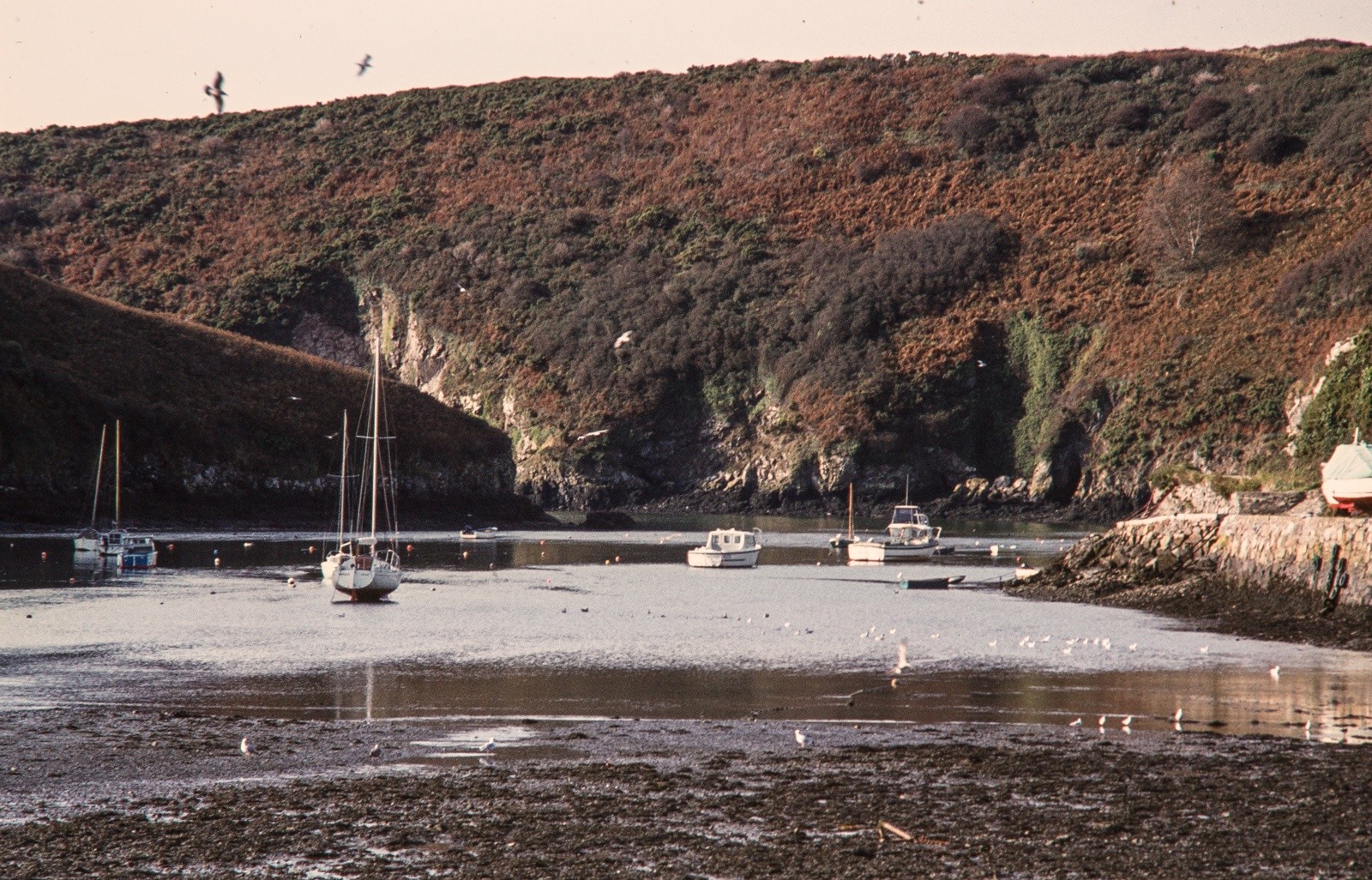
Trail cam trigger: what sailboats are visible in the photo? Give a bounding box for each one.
[73,424,107,551]
[321,326,403,601]
[74,550,100,565]
[99,420,127,554]
[98,554,123,569]
[828,482,862,551]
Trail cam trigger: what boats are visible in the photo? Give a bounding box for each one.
[1319,426,1372,514]
[688,526,764,569]
[847,535,940,562]
[885,473,942,542]
[898,577,949,591]
[122,533,158,554]
[122,552,157,571]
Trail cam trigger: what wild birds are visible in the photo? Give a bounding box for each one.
[25,528,1314,757]
[613,331,632,347]
[356,54,372,74]
[205,70,228,114]
